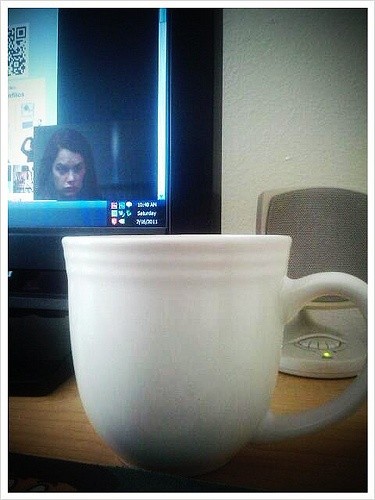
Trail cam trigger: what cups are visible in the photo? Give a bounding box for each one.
[59,233,367,480]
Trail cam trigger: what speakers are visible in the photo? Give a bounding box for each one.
[254,186,368,378]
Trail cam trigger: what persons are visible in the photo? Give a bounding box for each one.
[35,127,102,202]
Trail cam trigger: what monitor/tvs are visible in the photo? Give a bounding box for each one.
[8,8,224,313]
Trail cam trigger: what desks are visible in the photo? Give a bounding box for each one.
[8,369,368,493]
